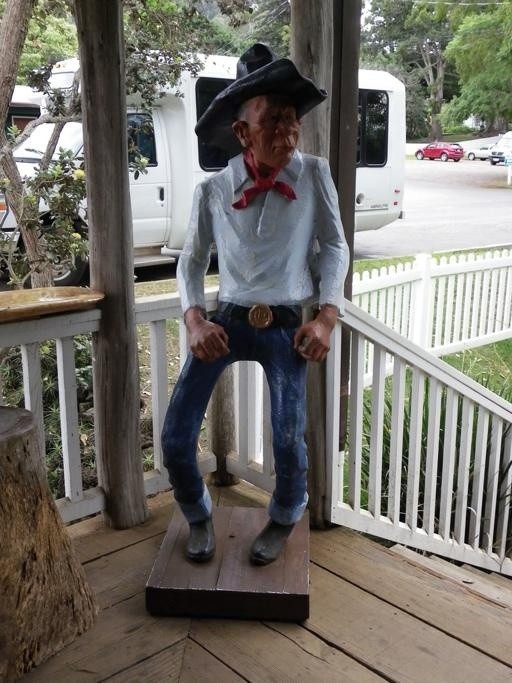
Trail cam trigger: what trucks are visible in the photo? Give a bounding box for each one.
[0,50,409,289]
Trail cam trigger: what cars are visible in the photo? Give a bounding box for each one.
[463,144,494,161]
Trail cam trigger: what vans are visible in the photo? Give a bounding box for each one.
[488,131,512,166]
[414,142,464,162]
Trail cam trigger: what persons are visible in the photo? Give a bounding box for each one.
[160,40,350,564]
